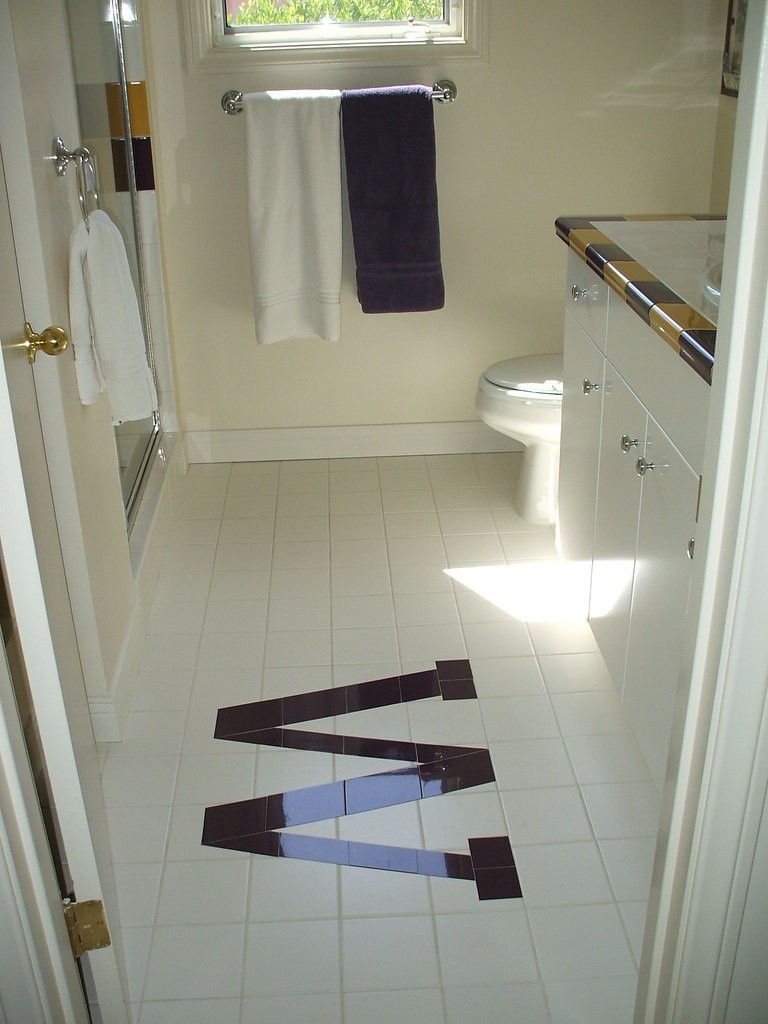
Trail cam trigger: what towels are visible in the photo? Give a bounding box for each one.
[241,88,342,344]
[69,208,160,428]
[340,84,446,313]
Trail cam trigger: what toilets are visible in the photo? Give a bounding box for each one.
[477,352,564,526]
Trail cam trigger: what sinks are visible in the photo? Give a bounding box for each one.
[699,259,722,307]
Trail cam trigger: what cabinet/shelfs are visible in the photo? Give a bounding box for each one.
[554,243,712,794]
